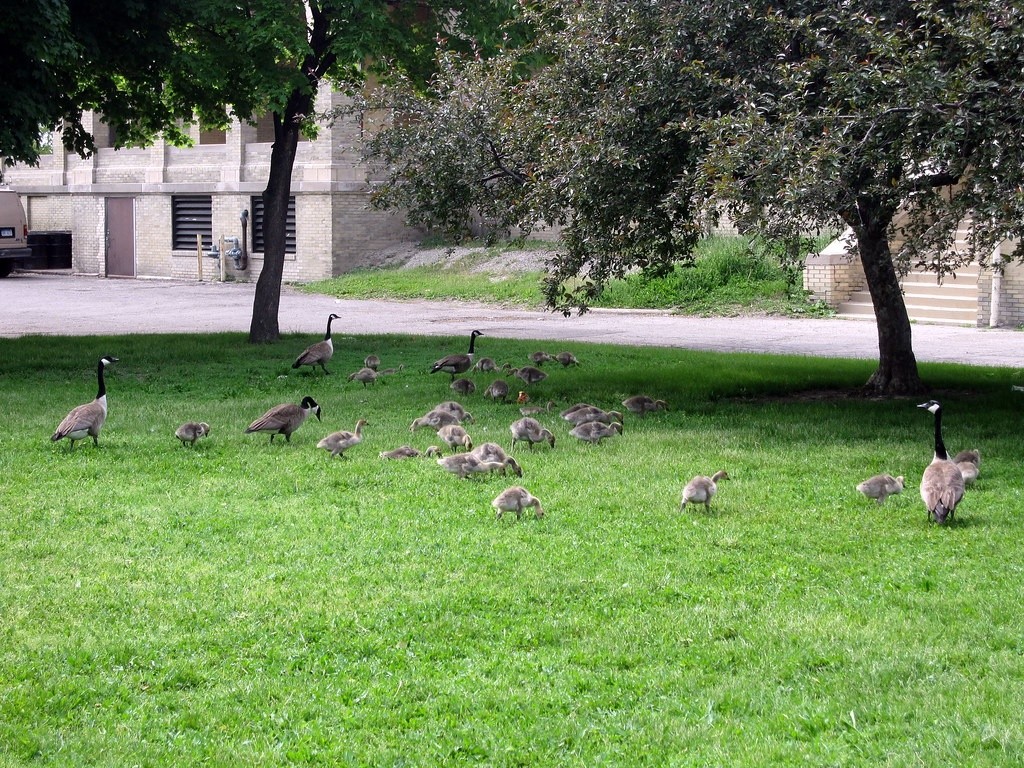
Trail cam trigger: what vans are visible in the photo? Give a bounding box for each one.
[0,189,32,277]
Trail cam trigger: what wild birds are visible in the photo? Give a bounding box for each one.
[917,400,965,525]
[856,474,905,503]
[679,470,731,512]
[317,419,369,460]
[492,486,544,520]
[380,329,671,478]
[175,422,210,447]
[953,449,980,465]
[347,369,378,388]
[51,355,120,450]
[244,396,321,442]
[292,314,341,375]
[377,364,404,375]
[956,461,979,488]
[364,355,380,370]
